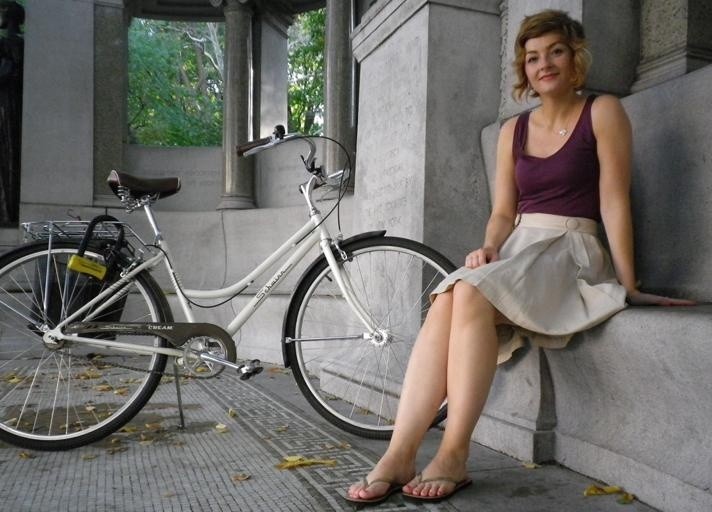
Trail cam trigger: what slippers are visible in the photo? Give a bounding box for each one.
[343,474,407,507]
[402,471,473,504]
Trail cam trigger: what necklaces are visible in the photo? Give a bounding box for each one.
[541,100,571,136]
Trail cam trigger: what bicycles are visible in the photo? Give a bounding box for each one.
[0,125,459,453]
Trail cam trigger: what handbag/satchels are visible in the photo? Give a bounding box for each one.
[28,253,128,342]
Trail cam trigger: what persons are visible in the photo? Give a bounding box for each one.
[345,10,698,502]
